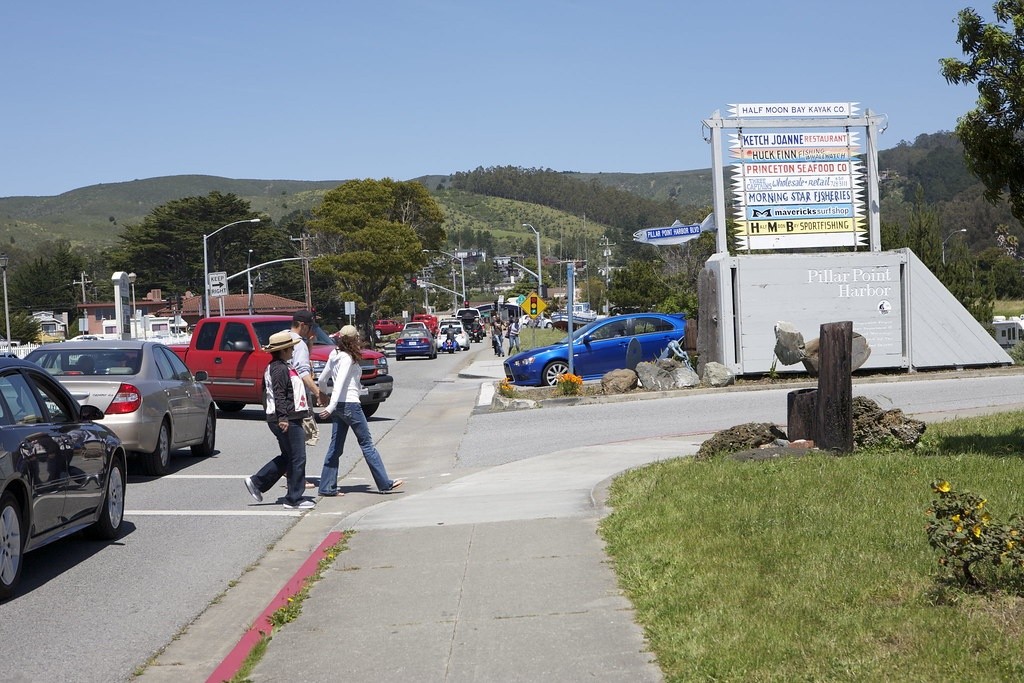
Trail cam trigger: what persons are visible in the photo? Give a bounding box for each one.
[471,318,482,343]
[444,324,461,351]
[317,325,403,496]
[490,315,506,357]
[284,310,327,490]
[611,323,625,338]
[509,316,513,324]
[506,318,521,356]
[245,332,316,509]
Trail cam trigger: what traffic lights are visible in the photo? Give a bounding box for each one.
[411,277,417,289]
[522,291,548,319]
[464,300,470,308]
[493,260,501,275]
[506,260,513,272]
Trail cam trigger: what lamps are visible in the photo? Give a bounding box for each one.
[701,124,711,145]
[868,112,889,134]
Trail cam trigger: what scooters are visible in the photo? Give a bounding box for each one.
[473,329,482,343]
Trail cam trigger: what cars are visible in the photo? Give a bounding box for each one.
[66,334,111,343]
[503,312,689,387]
[404,322,428,330]
[413,302,488,349]
[375,320,406,337]
[396,329,439,361]
[20,342,221,477]
[0,354,126,596]
[517,314,554,330]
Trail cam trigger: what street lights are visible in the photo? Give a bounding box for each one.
[201,218,266,320]
[942,228,967,266]
[246,249,258,316]
[128,272,140,340]
[0,252,14,353]
[523,223,544,298]
[423,248,467,309]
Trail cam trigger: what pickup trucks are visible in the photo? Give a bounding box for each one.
[0,336,21,349]
[106,316,393,421]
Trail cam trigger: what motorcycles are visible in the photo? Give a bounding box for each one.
[443,338,460,354]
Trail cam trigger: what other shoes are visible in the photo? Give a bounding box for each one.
[305,481,315,488]
[318,490,346,496]
[382,479,401,493]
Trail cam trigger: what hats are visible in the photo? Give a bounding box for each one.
[261,331,301,352]
[293,310,318,324]
[339,325,358,339]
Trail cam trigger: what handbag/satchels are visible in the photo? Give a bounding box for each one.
[301,416,319,446]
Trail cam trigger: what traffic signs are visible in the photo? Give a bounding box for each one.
[208,271,228,298]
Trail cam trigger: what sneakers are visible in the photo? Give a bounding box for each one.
[283,500,315,509]
[244,477,263,502]
